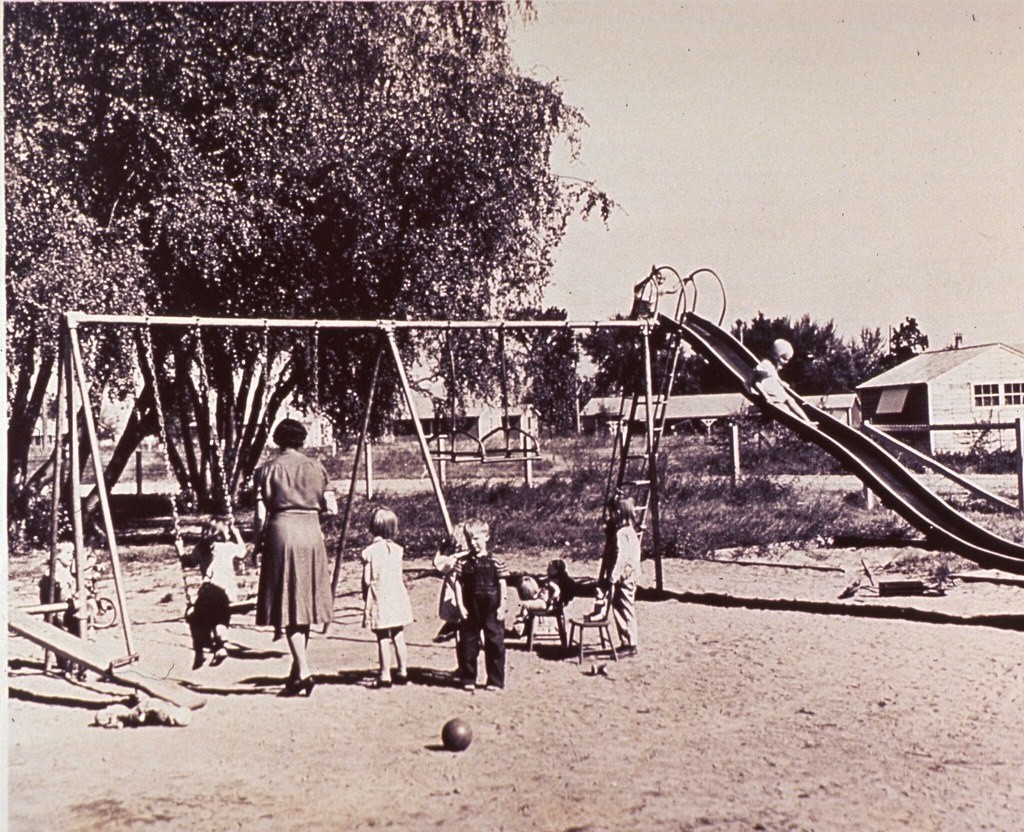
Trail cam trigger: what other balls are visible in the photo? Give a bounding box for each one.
[441,718,473,751]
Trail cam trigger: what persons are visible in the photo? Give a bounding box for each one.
[173,515,247,671]
[436,492,643,692]
[38,540,97,646]
[250,419,339,694]
[359,508,413,688]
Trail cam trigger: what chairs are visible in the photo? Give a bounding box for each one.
[527,572,568,652]
[567,584,619,665]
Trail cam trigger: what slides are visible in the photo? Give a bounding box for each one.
[657,309,1024,573]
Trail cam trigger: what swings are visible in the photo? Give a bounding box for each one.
[141,325,257,624]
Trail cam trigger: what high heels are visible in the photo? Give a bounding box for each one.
[278,675,316,697]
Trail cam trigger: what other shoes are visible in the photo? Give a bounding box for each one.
[391,668,409,686]
[208,649,227,666]
[366,672,392,687]
[610,645,637,660]
[192,652,206,670]
